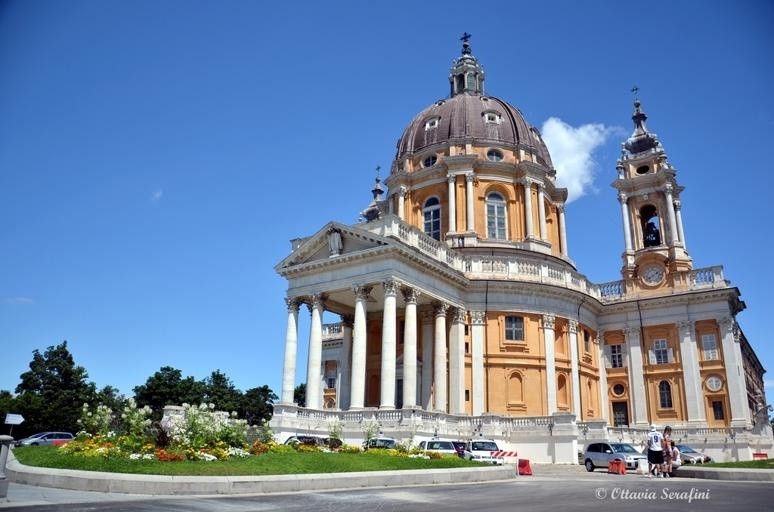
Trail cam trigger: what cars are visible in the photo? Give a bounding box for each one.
[417,440,504,466]
[283,435,396,449]
[584,443,711,472]
[16,432,74,447]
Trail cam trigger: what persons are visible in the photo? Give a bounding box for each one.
[647,424,681,478]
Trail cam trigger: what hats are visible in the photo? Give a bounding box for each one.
[651,424,657,432]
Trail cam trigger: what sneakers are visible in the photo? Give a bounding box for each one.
[647,470,675,478]
